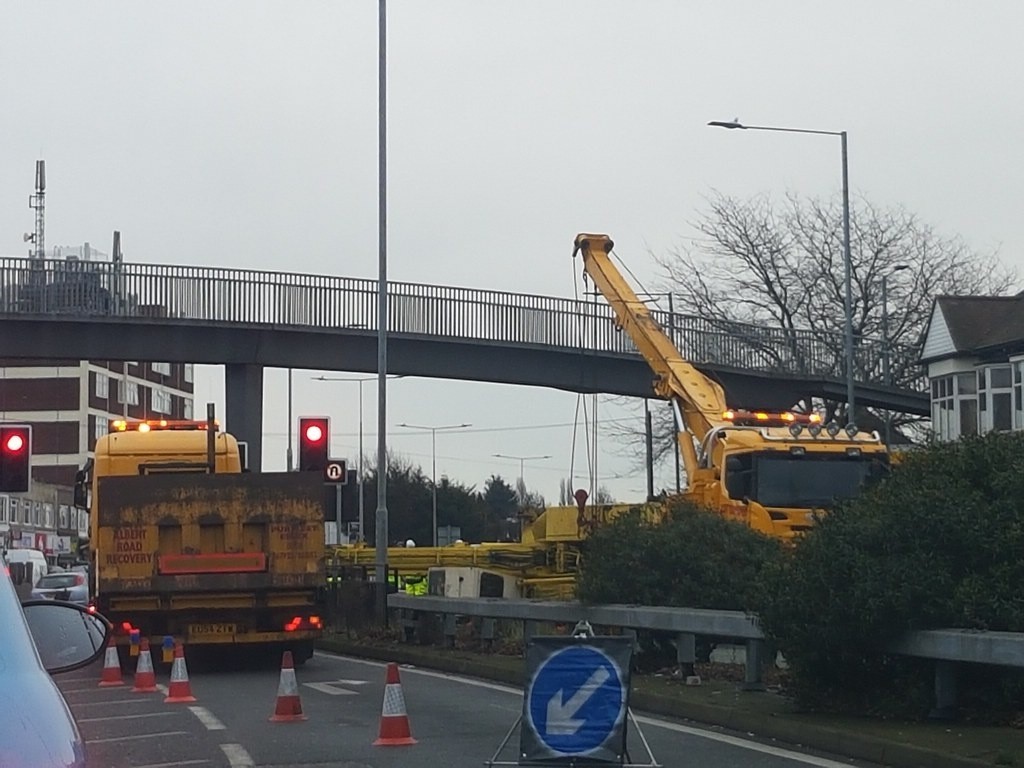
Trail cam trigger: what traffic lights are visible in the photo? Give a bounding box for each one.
[1,425,31,494]
[298,417,329,485]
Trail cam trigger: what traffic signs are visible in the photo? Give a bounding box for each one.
[525,635,630,767]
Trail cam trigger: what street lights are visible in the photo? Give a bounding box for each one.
[711,116,860,433]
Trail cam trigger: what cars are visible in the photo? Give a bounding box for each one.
[0,526,112,768]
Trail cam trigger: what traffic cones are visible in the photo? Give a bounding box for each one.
[371,664,419,745]
[268,650,308,722]
[162,639,198,702]
[99,634,123,688]
[130,639,158,694]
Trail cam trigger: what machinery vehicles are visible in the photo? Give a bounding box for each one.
[334,232,903,621]
[77,411,327,670]
